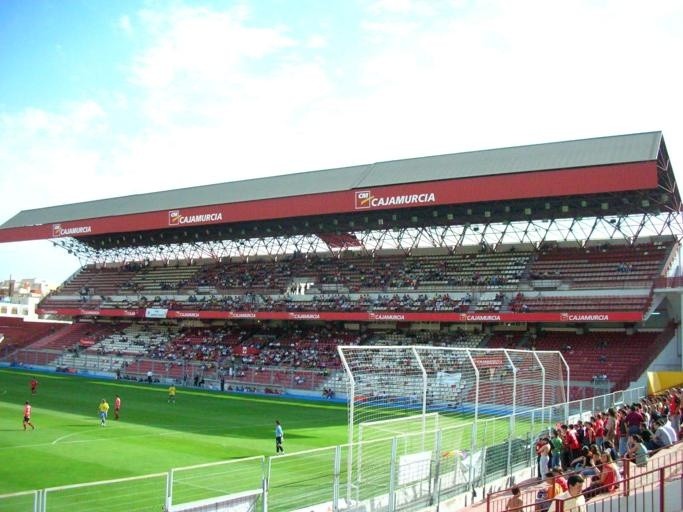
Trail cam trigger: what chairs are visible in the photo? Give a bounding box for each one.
[28,244,669,404]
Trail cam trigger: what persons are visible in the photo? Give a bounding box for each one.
[97,398,110,426]
[48,242,642,410]
[29,376,39,400]
[21,402,36,431]
[113,393,122,419]
[274,419,285,456]
[505,385,683,512]
